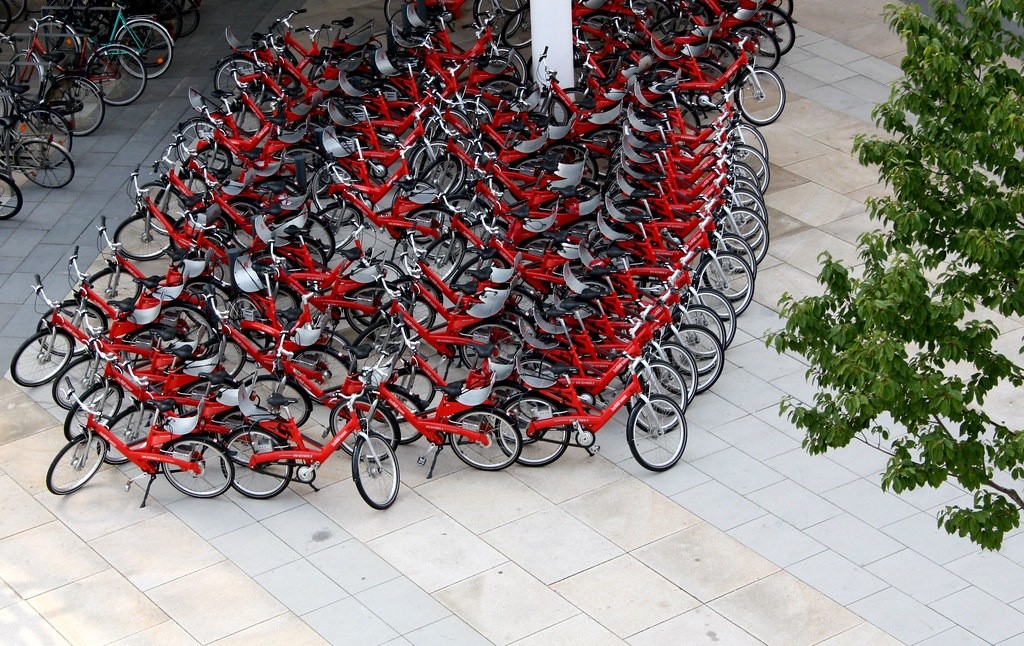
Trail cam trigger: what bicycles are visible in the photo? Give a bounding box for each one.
[0,1,803,511]
